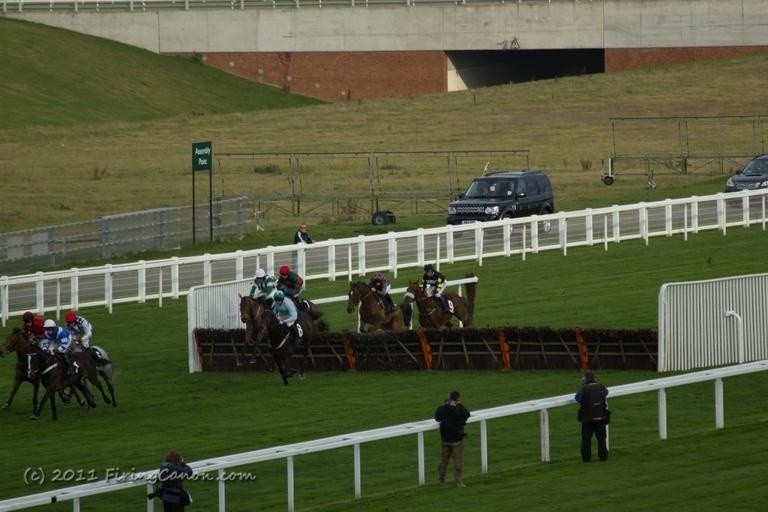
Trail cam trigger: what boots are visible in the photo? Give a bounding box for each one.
[85,348,101,362]
[439,294,450,313]
[61,353,75,375]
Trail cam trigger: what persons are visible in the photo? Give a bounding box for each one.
[23,310,93,374]
[575,371,611,463]
[251,264,447,333]
[158,450,193,512]
[434,390,471,487]
[292,224,313,273]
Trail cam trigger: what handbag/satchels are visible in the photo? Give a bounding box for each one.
[577,406,611,425]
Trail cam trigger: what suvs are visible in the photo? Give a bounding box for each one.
[441,167,555,237]
[722,152,767,208]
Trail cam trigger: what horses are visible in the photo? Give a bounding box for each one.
[259,304,312,388]
[244,308,323,345]
[81,341,114,411]
[404,278,470,329]
[1,326,44,419]
[346,280,406,333]
[236,292,317,324]
[22,339,112,420]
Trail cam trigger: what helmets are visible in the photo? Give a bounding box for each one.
[424,264,434,271]
[65,312,77,324]
[256,268,266,278]
[372,272,384,283]
[43,319,57,328]
[279,266,290,275]
[274,290,284,301]
[23,312,33,323]
